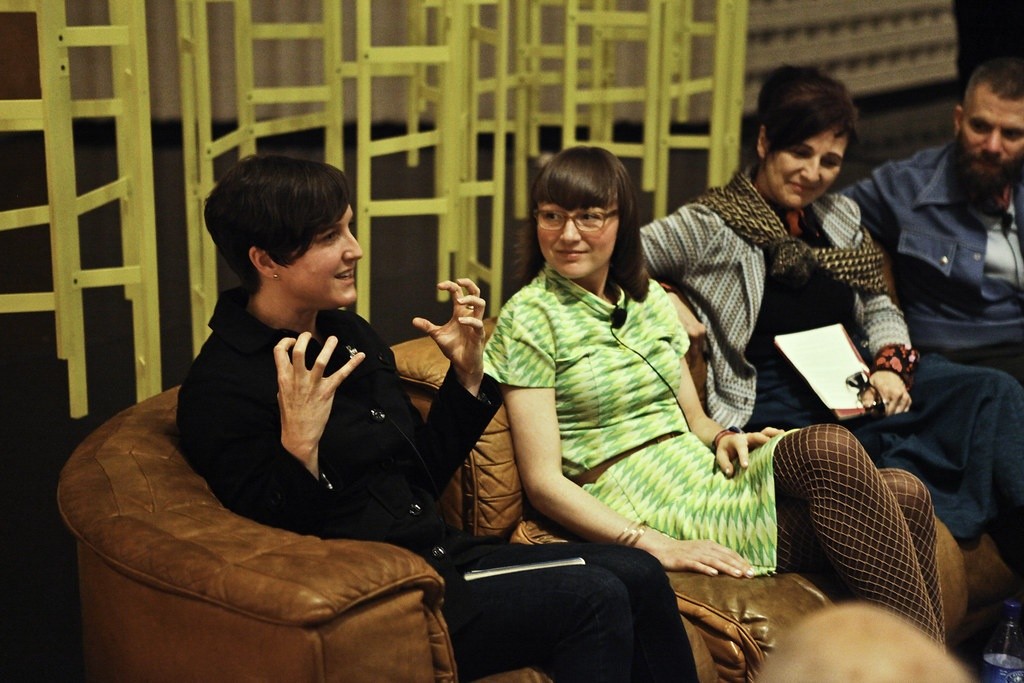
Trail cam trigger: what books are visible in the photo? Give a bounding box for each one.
[774,323,886,421]
[464,556,586,581]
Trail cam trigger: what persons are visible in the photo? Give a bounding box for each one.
[177,156,699,683]
[483,146,947,653]
[641,66,1024,575]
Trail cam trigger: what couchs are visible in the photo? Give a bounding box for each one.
[56,245,1019,683]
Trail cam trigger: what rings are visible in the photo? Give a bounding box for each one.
[466,305,474,309]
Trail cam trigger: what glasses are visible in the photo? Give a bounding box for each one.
[532,209,620,231]
[845,372,885,420]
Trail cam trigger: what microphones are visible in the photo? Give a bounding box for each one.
[609,308,628,329]
[1000,212,1013,237]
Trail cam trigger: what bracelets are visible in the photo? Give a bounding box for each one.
[712,425,741,449]
[616,520,647,547]
